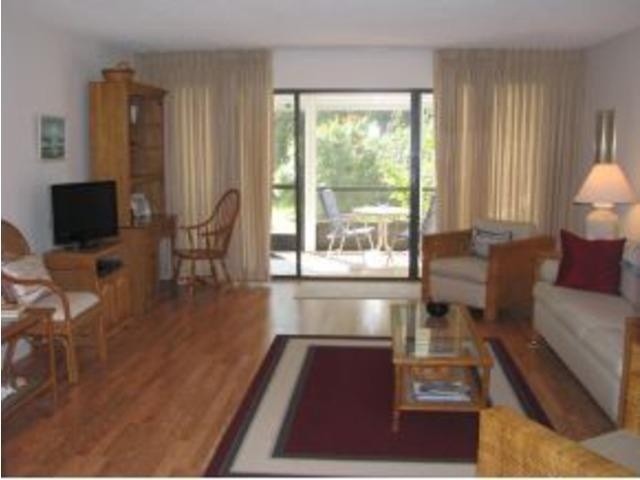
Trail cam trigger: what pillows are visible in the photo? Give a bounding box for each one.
[4,252,55,309]
[554,226,626,297]
[466,227,513,262]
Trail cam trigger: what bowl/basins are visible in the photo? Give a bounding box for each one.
[426,303,449,316]
[103,68,134,81]
[426,317,448,329]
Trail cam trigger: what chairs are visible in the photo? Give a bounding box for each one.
[385,195,440,267]
[317,187,374,263]
[2,217,107,381]
[170,189,243,296]
[477,314,640,477]
[419,216,558,321]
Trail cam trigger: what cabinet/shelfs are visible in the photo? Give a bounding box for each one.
[45,237,135,344]
[87,80,169,226]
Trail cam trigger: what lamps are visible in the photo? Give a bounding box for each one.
[571,164,636,237]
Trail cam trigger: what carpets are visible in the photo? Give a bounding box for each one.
[196,333,555,477]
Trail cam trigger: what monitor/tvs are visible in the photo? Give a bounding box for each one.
[51,180,118,249]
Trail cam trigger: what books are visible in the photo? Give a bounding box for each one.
[408,340,472,402]
[2,302,26,321]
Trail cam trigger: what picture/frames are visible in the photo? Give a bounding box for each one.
[36,112,68,164]
[128,192,152,218]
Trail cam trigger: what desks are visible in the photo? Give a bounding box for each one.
[112,213,178,308]
[353,202,413,264]
[0,304,58,427]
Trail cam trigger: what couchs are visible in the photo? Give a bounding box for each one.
[523,232,640,428]
